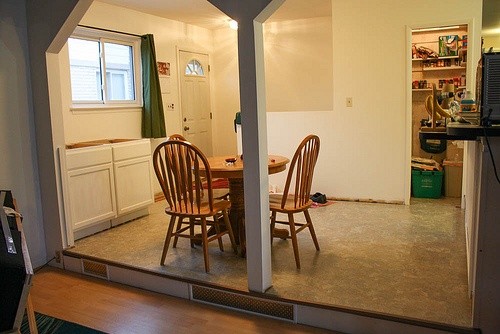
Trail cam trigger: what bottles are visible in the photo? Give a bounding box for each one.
[461,75,466,86]
[412,44,416,59]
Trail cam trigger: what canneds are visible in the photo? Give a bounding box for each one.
[423,60,447,68]
[415,80,427,89]
[439,79,453,88]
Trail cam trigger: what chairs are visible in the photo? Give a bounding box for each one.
[270,135,320,270]
[153,140,237,273]
[164,134,226,252]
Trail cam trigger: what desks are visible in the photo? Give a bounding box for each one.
[190,153,289,245]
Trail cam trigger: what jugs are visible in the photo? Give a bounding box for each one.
[234,112,242,157]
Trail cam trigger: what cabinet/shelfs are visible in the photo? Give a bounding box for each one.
[412,24,469,162]
[66,138,154,241]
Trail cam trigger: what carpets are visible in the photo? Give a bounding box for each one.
[20,307,109,334]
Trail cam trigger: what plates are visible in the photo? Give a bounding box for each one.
[226,158,237,163]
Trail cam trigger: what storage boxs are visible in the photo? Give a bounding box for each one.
[413,168,444,199]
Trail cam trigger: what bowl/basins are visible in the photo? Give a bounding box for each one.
[461,99,474,111]
[426,122,442,127]
